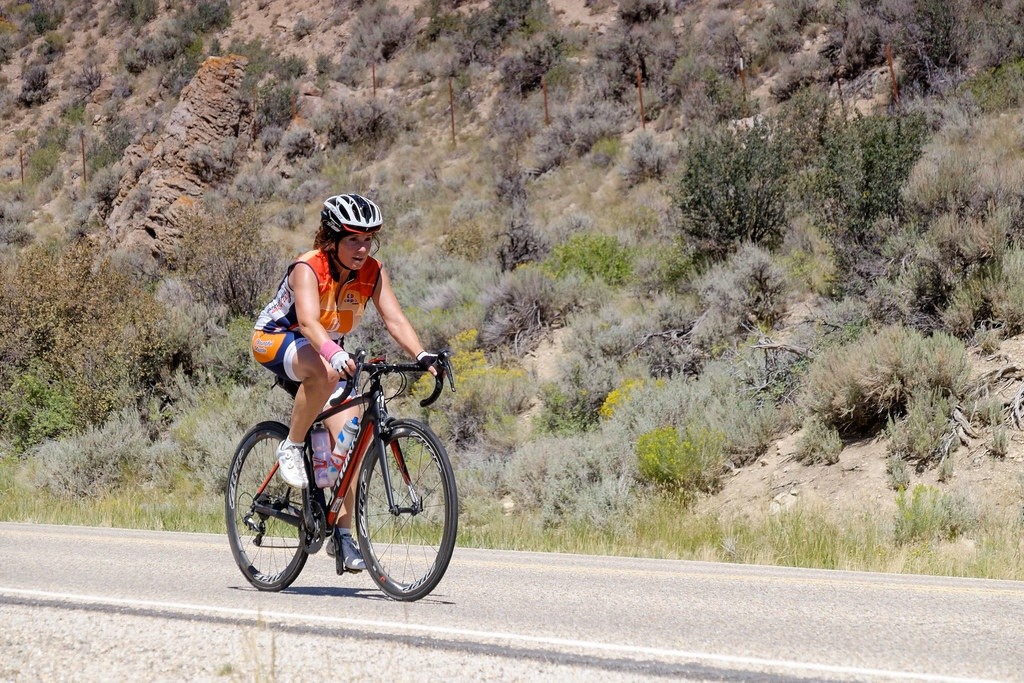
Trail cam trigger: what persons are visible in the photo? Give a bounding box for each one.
[250,193,447,570]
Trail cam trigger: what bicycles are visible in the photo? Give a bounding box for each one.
[225,348,458,602]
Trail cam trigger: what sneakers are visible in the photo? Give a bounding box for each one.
[326,533,373,570]
[275,435,309,489]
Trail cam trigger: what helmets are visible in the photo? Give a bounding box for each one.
[320,193,384,235]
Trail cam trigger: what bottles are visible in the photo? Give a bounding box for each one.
[311,423,335,488]
[326,417,360,479]
